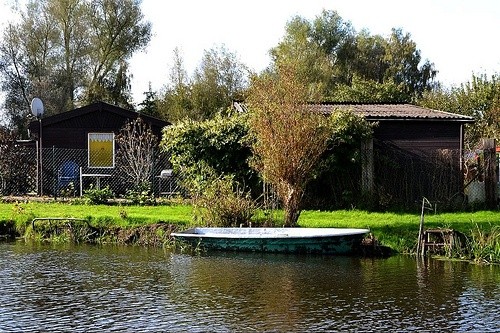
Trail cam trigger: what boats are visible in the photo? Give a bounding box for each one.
[170,225,371,255]
